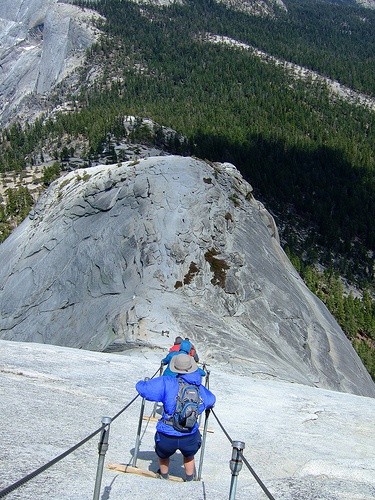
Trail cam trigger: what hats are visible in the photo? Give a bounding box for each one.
[169,353,199,374]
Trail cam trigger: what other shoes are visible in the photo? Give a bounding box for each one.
[181,472,194,482]
[156,468,169,480]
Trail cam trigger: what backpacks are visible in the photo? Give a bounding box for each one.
[159,374,203,433]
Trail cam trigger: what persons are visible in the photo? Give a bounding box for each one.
[136,354,216,482]
[162,341,206,377]
[170,337,195,356]
